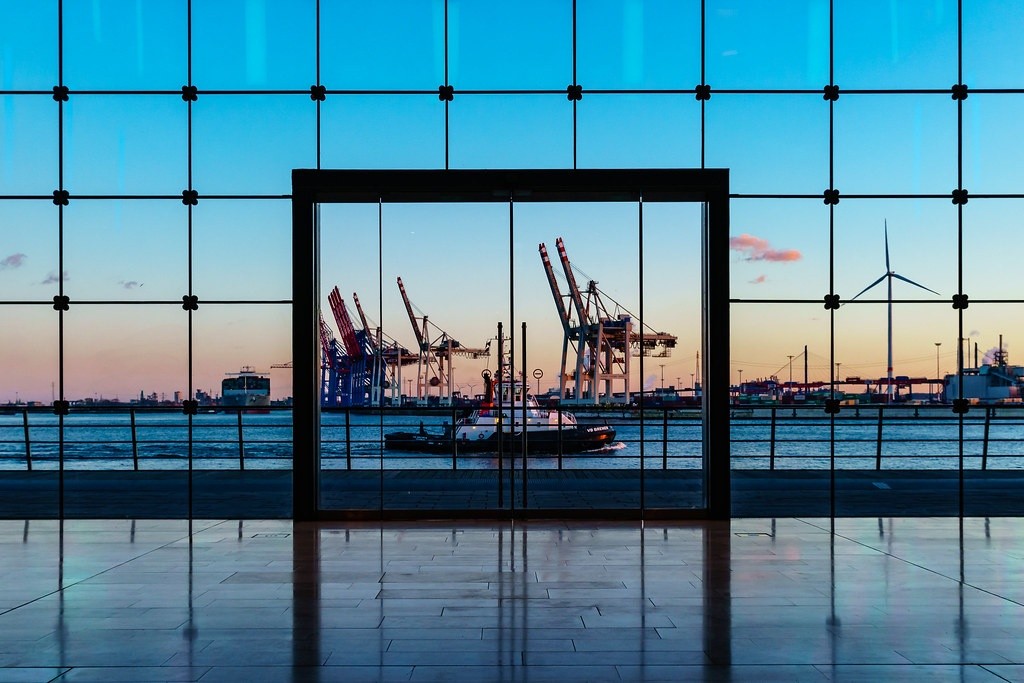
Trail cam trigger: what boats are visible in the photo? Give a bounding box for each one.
[385,335,616,456]
[221,365,270,414]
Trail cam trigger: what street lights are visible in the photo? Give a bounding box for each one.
[659,365,666,396]
[935,343,941,393]
[690,373,695,396]
[676,377,680,389]
[836,363,842,391]
[737,370,743,383]
[787,355,795,395]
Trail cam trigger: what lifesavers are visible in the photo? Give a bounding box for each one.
[478,434,484,439]
